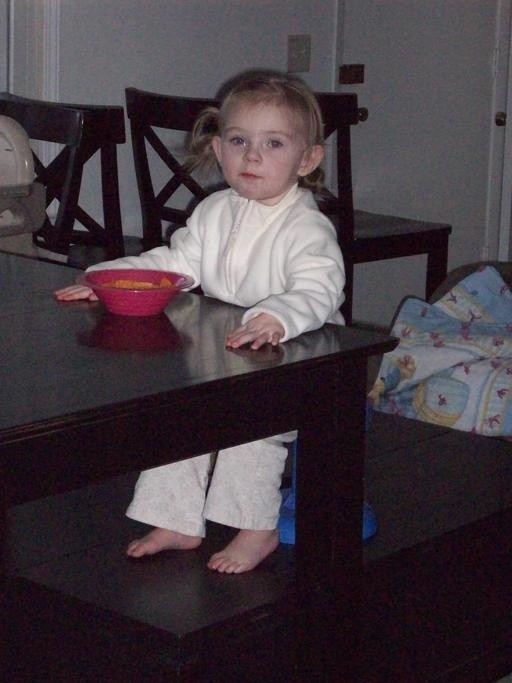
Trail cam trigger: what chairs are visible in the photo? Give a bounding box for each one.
[0,88,452,331]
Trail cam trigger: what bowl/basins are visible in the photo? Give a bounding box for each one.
[74,268,195,318]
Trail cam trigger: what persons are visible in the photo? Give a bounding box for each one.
[51,67,350,577]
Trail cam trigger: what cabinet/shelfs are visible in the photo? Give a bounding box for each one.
[0,401,512,683]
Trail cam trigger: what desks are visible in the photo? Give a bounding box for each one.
[1,254,402,681]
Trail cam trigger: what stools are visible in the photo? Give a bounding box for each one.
[275,405,381,549]
